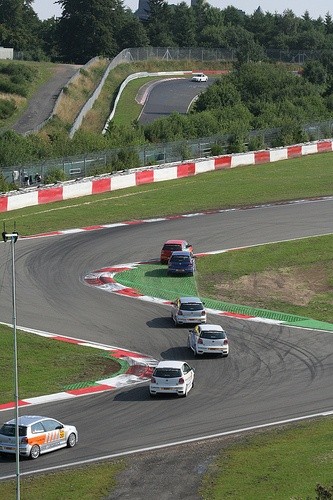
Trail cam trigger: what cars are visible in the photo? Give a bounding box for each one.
[149,360,195,398]
[0,415,78,460]
[170,296,206,327]
[168,251,196,276]
[161,240,192,265]
[187,324,229,358]
[192,73,209,82]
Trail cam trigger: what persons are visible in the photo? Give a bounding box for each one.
[22,168,28,182]
[29,175,32,186]
[34,173,42,182]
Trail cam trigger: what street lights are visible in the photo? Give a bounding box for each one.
[2,231,21,500]
